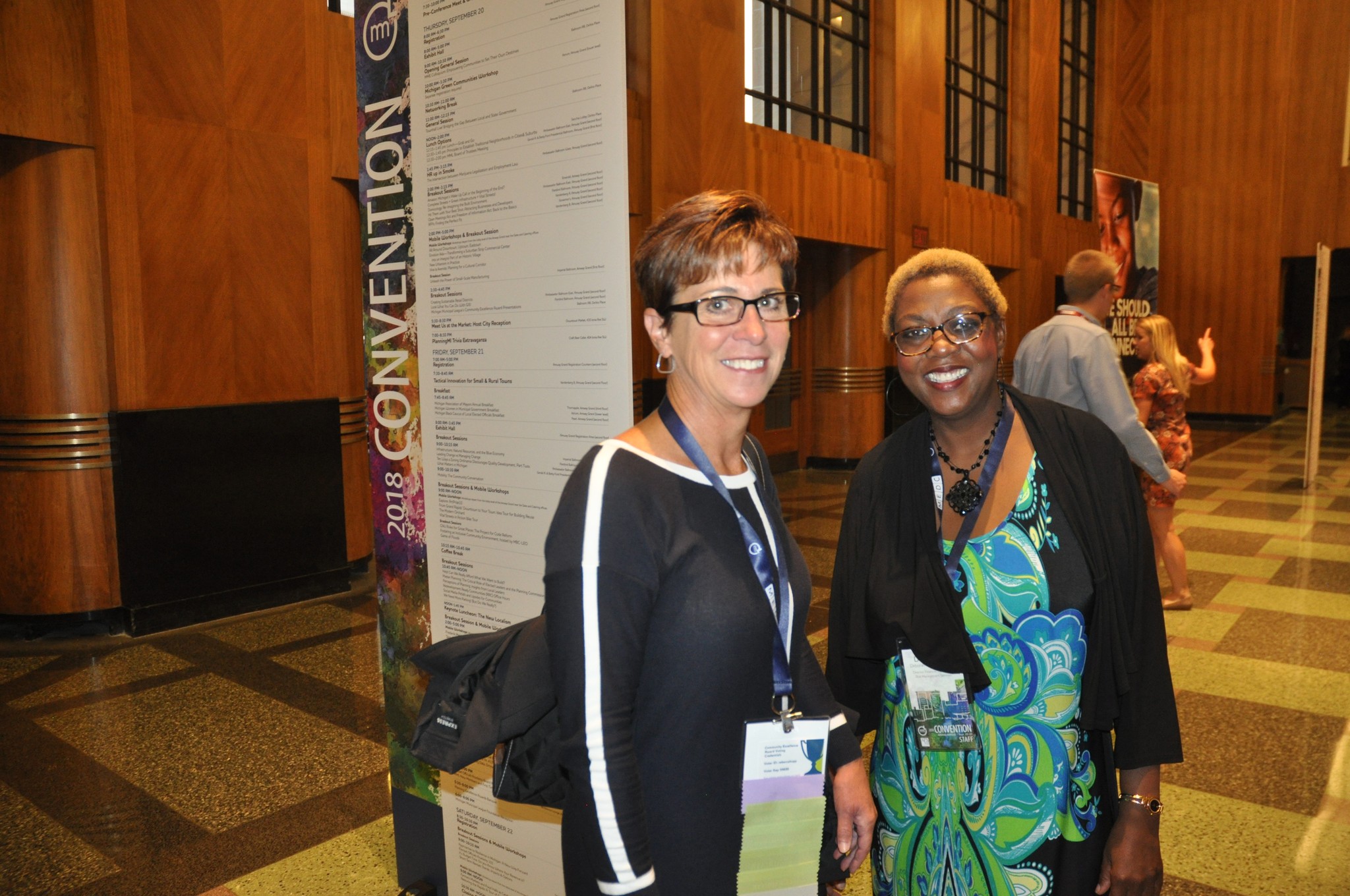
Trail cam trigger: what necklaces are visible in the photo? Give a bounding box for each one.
[928,396,1005,516]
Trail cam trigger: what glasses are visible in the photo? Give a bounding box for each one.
[669,292,800,327]
[1111,283,1121,292]
[890,311,997,356]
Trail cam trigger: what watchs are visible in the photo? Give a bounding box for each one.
[1118,794,1163,815]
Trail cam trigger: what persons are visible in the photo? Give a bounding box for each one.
[1012,249,1188,500]
[826,247,1184,896]
[1093,171,1158,382]
[1130,314,1217,611]
[542,188,878,896]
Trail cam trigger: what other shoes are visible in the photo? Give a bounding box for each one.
[1162,596,1192,611]
